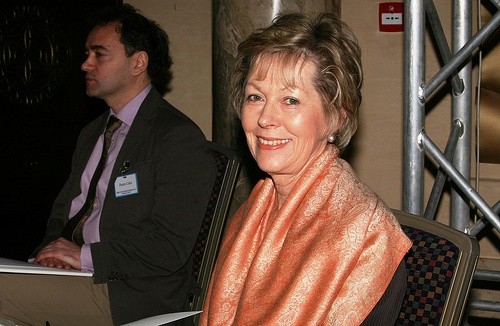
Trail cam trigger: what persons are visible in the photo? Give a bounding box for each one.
[1,3,218,326]
[196,10,414,326]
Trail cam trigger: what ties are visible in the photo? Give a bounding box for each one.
[62,115,123,245]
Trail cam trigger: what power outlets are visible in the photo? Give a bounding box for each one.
[378,1,404,32]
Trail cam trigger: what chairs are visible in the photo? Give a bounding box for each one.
[184,141,244,326]
[387,208,482,326]
